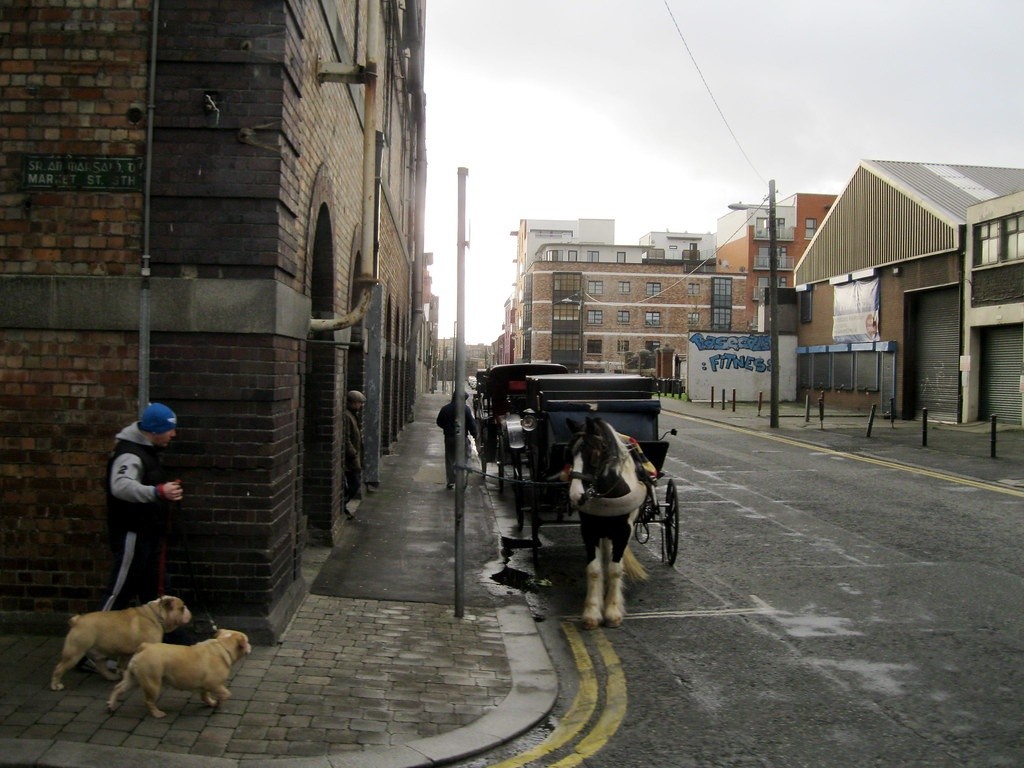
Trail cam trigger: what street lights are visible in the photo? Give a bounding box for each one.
[728,203,780,429]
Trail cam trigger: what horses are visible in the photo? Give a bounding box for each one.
[565,416,650,630]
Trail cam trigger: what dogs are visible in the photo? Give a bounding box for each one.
[106,629,251,718]
[50,596,192,690]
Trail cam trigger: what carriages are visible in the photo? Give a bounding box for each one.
[471,359,679,631]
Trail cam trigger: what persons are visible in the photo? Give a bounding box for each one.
[438,390,481,489]
[81,404,179,681]
[343,391,367,518]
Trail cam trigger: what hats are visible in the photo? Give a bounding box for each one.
[140,402,178,432]
[346,390,368,402]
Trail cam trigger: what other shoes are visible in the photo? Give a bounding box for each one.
[84,657,121,680]
